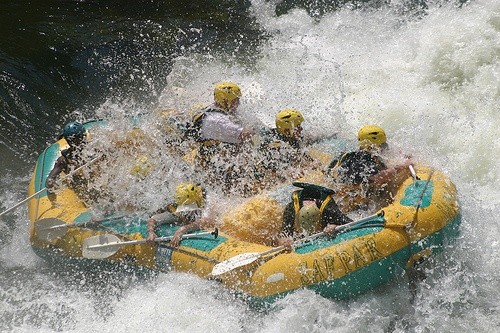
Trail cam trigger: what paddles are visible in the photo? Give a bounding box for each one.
[81,227,218,259]
[33,211,139,242]
[0,153,103,217]
[409,165,420,181]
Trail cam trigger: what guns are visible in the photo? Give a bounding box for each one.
[212,209,385,275]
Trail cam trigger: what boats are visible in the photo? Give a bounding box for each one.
[28,103,461,315]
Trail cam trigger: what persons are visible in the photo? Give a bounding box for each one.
[280,181,353,252]
[251,108,338,183]
[180,82,256,167]
[146,181,212,246]
[333,125,415,214]
[45,121,106,202]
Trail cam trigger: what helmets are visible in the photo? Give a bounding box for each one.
[173,184,203,207]
[298,204,321,233]
[358,125,385,149]
[213,82,241,107]
[63,121,86,141]
[275,109,304,138]
